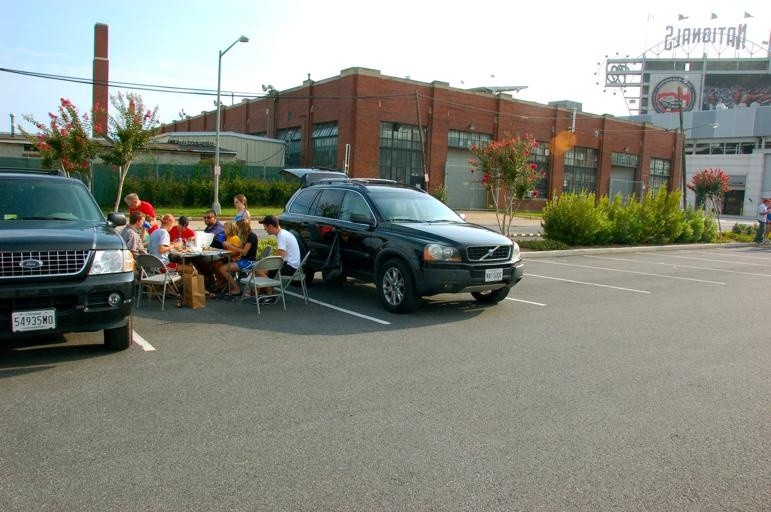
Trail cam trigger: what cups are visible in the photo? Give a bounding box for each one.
[218,234,225,240]
[143,221,150,228]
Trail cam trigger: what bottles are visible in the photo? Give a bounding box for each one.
[176,292,182,307]
[177,237,184,249]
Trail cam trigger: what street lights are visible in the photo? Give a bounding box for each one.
[675,86,720,212]
[209,34,251,214]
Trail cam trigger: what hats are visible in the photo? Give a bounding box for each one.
[259,214,279,225]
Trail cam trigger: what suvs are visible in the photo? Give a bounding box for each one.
[1,165,134,355]
[275,164,526,313]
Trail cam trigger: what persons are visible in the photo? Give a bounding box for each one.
[755,199,771,242]
[120,193,301,305]
[707,86,761,110]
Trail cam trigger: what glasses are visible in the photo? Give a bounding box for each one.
[202,215,216,220]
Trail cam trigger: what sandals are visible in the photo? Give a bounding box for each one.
[224,292,242,297]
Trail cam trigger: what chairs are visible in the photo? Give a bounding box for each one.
[128,235,311,316]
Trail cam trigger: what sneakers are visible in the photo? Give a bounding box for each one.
[254,298,273,305]
